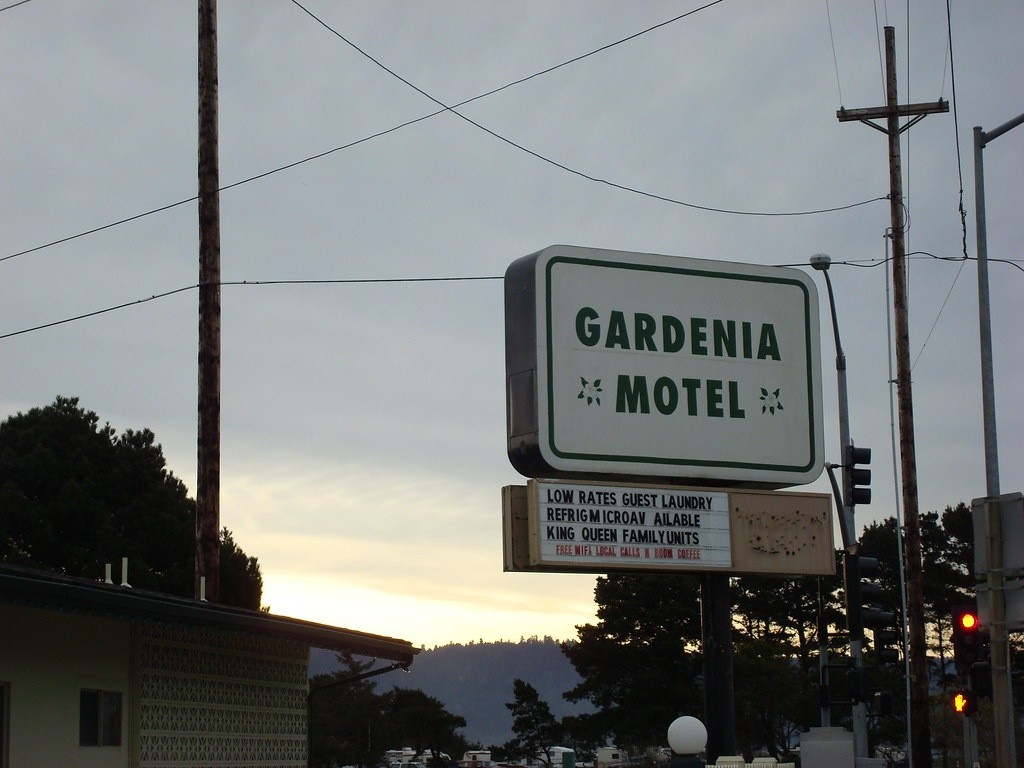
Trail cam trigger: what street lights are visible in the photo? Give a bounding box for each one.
[810,253,877,680]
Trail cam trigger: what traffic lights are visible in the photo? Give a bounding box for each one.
[873,612,900,663]
[952,691,970,714]
[952,606,980,673]
[842,554,884,628]
[842,444,871,505]
